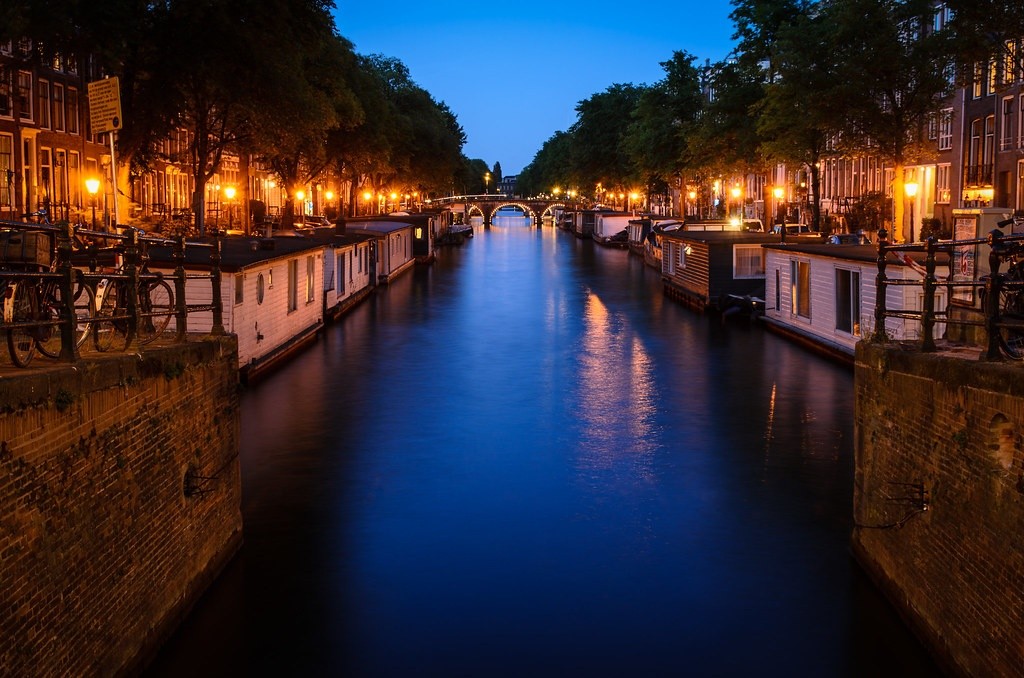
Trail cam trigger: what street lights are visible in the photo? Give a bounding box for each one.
[86,180,100,231]
[297,191,303,223]
[226,187,235,230]
[905,183,919,244]
[774,187,783,224]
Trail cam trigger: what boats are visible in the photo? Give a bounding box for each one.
[624,215,685,270]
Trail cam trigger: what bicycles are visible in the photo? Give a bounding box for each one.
[93,224,174,353]
[980,210,1024,361]
[0,210,96,369]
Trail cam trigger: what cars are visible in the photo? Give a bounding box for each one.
[773,224,809,234]
[831,234,871,245]
